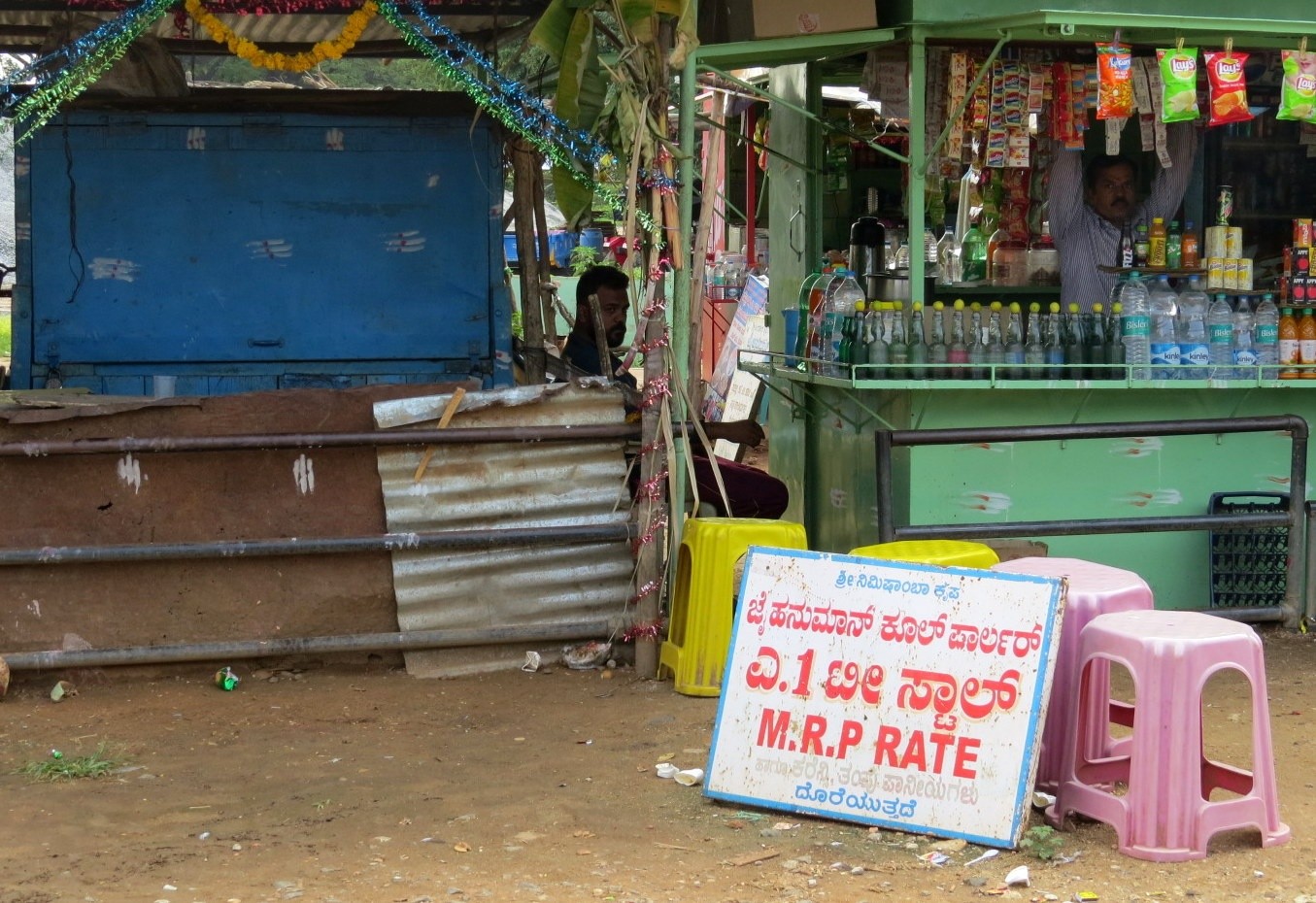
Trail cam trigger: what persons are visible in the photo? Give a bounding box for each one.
[1048,110,1198,314]
[553,266,789,520]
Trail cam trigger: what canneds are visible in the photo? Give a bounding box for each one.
[1215,185,1234,225]
[1197,226,1254,290]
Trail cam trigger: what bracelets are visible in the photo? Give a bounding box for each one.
[694,419,705,441]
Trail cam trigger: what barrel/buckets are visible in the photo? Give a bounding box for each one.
[862,268,936,305]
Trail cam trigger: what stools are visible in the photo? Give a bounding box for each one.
[658,518,808,698]
[848,540,1291,862]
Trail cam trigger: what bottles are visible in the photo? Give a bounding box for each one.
[1110,218,1316,381]
[883,222,1010,285]
[866,300,1125,381]
[796,266,869,378]
[703,244,750,300]
